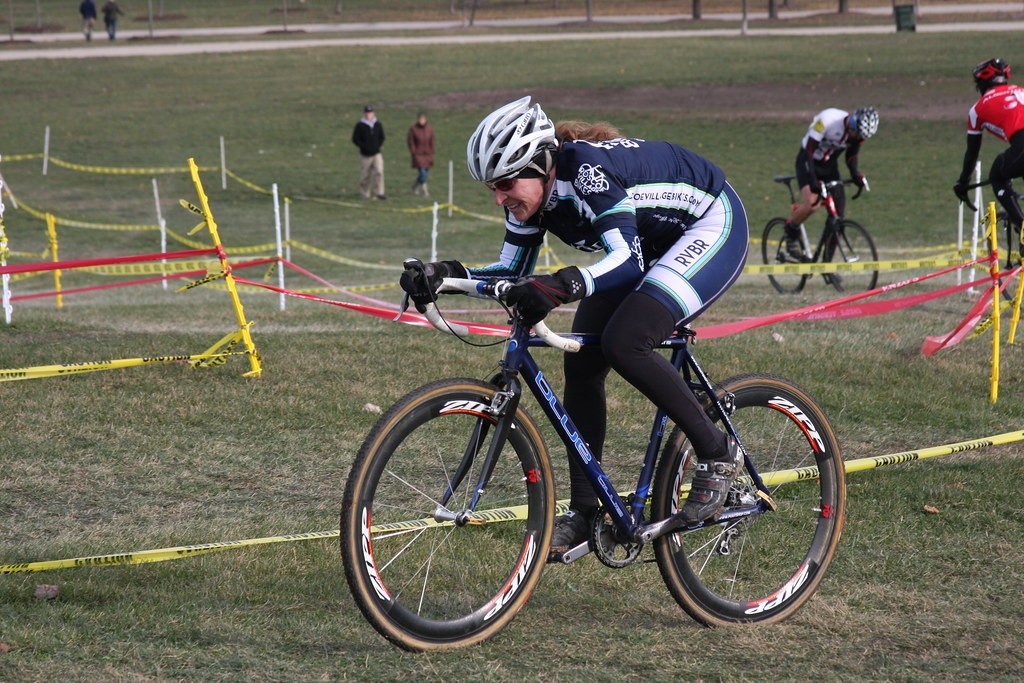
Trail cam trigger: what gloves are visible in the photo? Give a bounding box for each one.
[810,177,822,194]
[399,257,468,314]
[954,185,968,201]
[852,172,865,186]
[506,265,595,326]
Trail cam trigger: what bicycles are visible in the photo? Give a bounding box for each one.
[961,177,1024,317]
[760,175,880,296]
[339,256,847,654]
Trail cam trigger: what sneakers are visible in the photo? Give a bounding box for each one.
[551,509,606,546]
[784,224,802,259]
[682,433,744,523]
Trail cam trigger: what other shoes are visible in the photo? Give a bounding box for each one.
[378,194,386,200]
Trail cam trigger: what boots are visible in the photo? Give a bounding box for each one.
[421,184,430,196]
[412,179,421,195]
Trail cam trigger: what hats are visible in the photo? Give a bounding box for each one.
[364,105,374,112]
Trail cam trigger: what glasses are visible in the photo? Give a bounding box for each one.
[485,179,514,192]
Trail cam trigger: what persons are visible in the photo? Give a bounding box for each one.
[784,106,879,283]
[101,0,124,40]
[952,57,1024,258]
[408,112,435,197]
[352,105,386,200]
[399,96,749,552]
[79,0,97,41]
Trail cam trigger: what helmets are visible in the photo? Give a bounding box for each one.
[972,57,1011,83]
[466,96,556,183]
[856,105,880,140]
